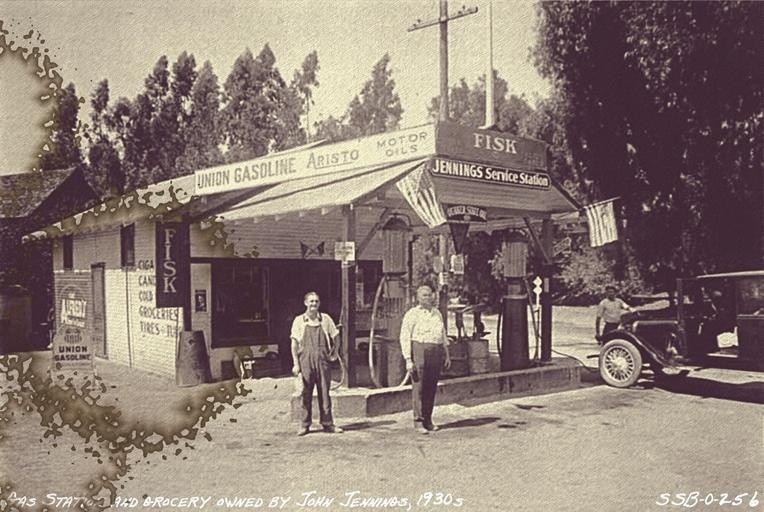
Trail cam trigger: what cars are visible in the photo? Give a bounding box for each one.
[589,267,763,398]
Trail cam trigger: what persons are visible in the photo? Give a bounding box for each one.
[290,290,343,437]
[595,285,637,342]
[399,285,452,434]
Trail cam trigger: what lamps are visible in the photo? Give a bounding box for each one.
[499,227,530,287]
[376,211,414,284]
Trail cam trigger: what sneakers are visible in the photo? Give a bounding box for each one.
[325,425,342,432]
[298,427,308,435]
[415,426,438,433]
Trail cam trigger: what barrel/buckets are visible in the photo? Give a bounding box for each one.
[441,341,469,379]
[468,339,490,374]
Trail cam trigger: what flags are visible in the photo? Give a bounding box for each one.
[585,202,619,247]
